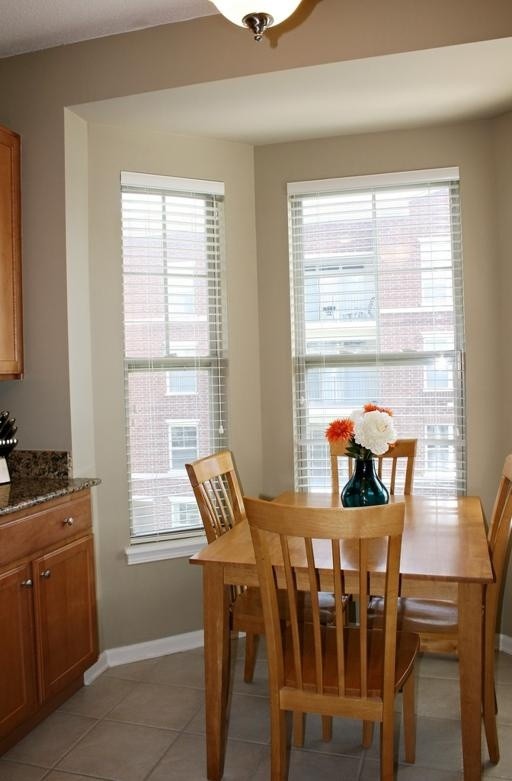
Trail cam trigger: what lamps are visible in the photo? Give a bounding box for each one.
[203,1,306,40]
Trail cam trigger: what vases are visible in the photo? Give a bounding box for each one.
[336,455,388,507]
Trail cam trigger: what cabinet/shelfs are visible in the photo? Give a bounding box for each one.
[1,126,24,380]
[1,495,99,738]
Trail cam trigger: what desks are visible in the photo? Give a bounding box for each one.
[189,498,496,777]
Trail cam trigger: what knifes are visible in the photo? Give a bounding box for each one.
[0,408,18,458]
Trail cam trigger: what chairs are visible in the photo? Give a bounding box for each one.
[240,495,418,781]
[326,436,421,493]
[187,449,332,740]
[356,451,512,761]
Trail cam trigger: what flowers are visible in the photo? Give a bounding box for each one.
[326,403,398,462]
